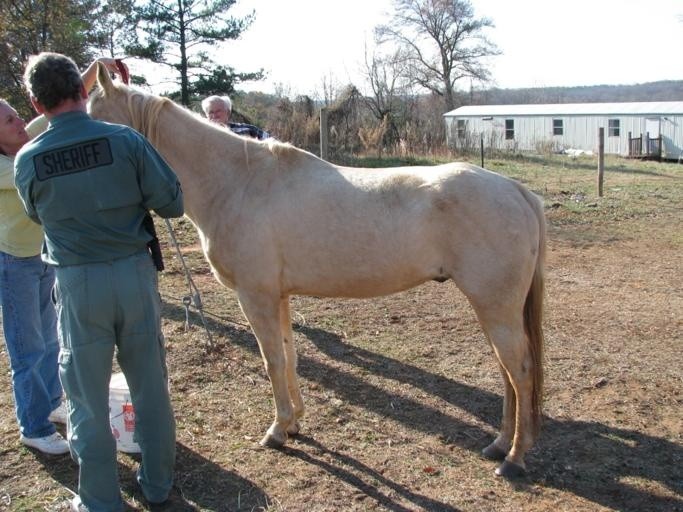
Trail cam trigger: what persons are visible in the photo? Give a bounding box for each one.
[0,56,130,455]
[13,51,184,512]
[200,93,272,141]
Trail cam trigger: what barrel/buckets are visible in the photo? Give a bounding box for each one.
[107,372,170,453]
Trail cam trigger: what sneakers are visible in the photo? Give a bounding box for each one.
[47,401,67,423]
[72,495,89,512]
[19,431,70,454]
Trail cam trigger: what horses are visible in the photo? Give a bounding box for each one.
[85,59,548,479]
